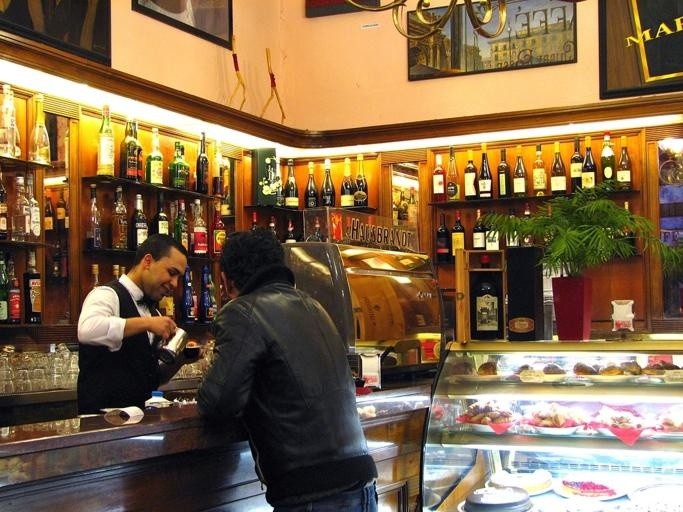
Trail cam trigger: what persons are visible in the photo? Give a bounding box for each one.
[198,230,379,512]
[78,233,189,417]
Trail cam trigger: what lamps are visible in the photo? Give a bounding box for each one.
[656,136,683,188]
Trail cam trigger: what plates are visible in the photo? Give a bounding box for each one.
[458,469,682,510]
[467,420,683,439]
[452,370,680,385]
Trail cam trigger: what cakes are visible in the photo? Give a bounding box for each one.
[504,470,552,492]
[561,480,616,496]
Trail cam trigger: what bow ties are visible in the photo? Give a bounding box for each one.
[137,295,160,314]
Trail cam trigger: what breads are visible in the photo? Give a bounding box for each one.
[466,363,678,428]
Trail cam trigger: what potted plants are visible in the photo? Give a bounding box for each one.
[473,181,683,340]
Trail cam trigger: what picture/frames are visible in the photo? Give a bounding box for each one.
[0,0,114,67]
[598,1,683,102]
[408,1,578,84]
[130,1,234,51]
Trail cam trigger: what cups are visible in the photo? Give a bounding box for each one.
[1,416,81,443]
[0,340,215,392]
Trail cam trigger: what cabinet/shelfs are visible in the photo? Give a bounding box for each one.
[1,151,646,329]
[417,333,683,512]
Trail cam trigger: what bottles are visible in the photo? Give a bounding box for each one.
[427,136,630,204]
[281,150,367,208]
[470,254,502,343]
[95,105,210,196]
[247,211,294,244]
[85,181,225,260]
[85,263,220,326]
[435,199,633,257]
[310,217,321,234]
[0,83,70,326]
